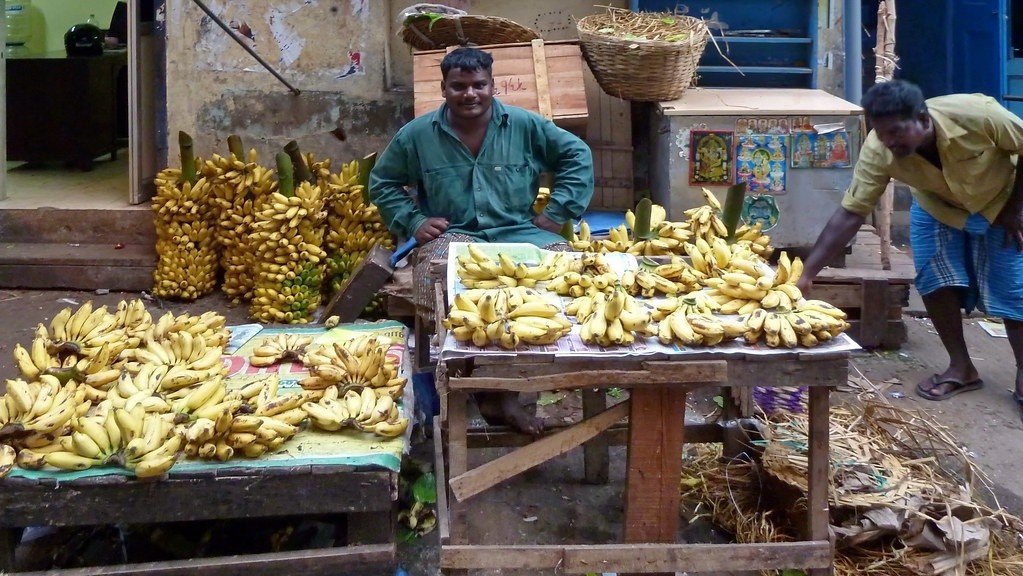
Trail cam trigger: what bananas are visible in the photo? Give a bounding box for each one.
[149,148,395,326]
[440,187,852,348]
[0,298,411,482]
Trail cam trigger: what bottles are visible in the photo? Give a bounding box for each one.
[5,41,29,56]
[5,0,32,43]
[86,14,99,27]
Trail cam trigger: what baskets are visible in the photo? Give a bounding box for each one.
[578,14,705,102]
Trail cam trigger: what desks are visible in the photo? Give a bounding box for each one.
[6,48,127,174]
[0,318,410,576]
[424,259,852,576]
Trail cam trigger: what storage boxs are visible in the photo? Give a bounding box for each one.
[643,87,865,252]
[411,41,588,134]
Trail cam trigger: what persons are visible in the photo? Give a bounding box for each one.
[797,81,1023,420]
[367,49,594,436]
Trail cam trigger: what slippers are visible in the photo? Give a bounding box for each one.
[916,372,983,400]
[1012,391,1023,410]
